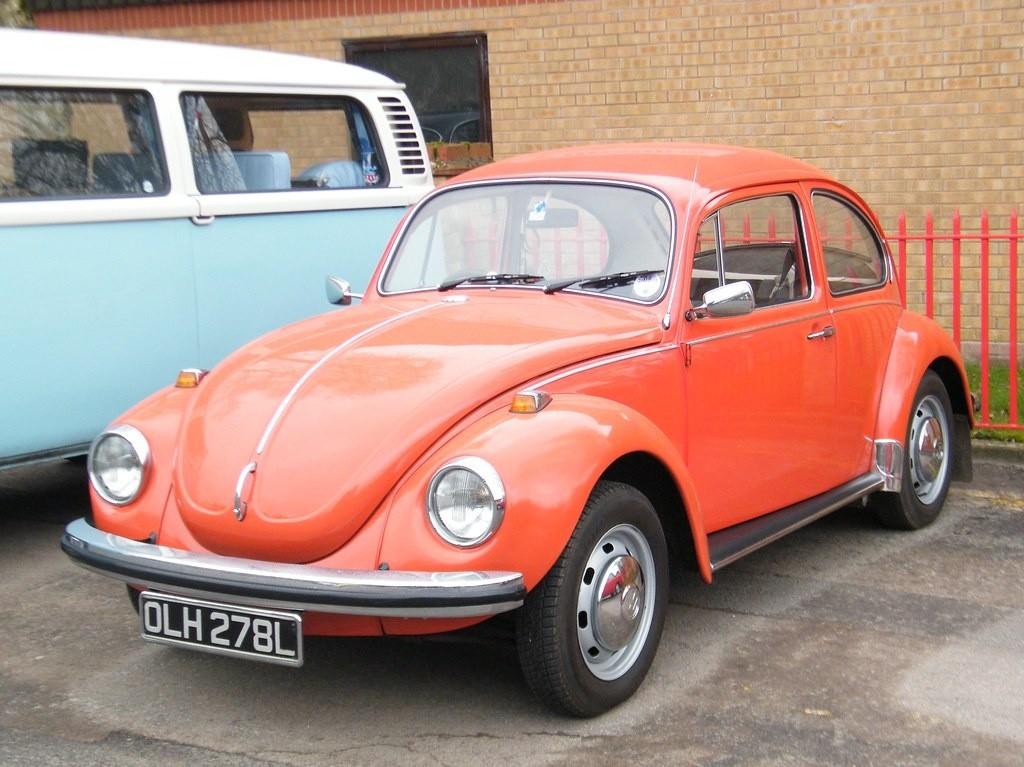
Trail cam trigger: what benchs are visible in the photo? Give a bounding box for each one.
[91,152,291,191]
[692,278,868,308]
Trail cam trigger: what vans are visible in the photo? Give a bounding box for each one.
[0,28,440,471]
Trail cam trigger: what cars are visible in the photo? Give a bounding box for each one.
[59,145,976,720]
